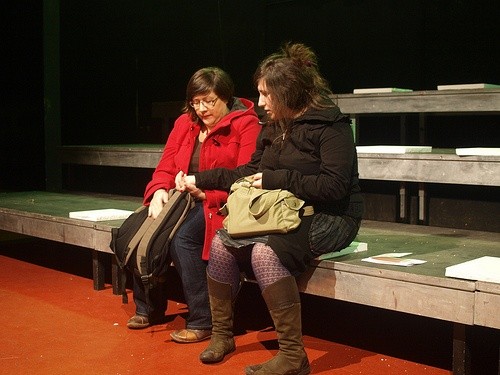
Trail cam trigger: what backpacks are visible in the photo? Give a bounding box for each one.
[109,185,193,294]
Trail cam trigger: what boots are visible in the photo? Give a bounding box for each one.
[245,276,310,375]
[200,263,237,363]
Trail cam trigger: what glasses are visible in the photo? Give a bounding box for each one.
[190,97,219,108]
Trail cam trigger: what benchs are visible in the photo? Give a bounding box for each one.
[0,88,500,375]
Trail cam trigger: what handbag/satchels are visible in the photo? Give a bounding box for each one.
[220,174,313,238]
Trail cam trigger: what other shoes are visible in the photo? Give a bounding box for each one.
[127,316,166,329]
[170,325,214,343]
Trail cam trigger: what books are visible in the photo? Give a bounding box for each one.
[456,148,500,156]
[356,146,432,153]
[69,209,134,221]
[314,241,367,260]
[446,256,500,283]
[354,88,413,93]
[438,84,500,90]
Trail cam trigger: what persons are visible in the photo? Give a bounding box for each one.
[126,67,264,342]
[176,44,363,375]
